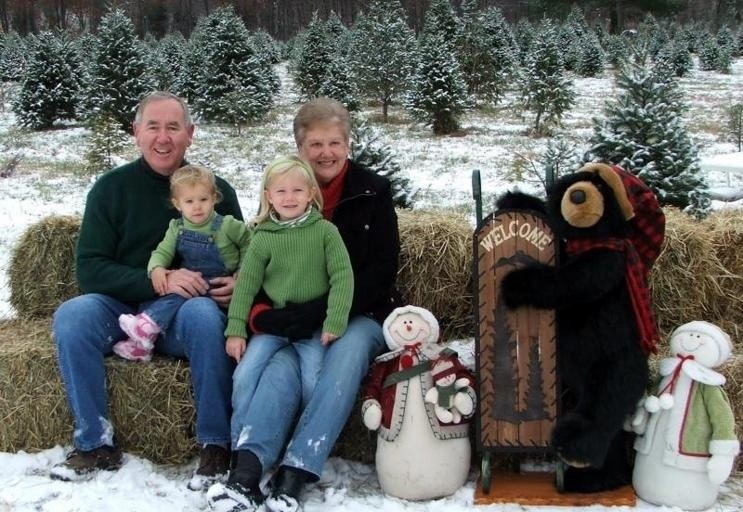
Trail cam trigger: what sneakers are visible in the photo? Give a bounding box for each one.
[118,311,161,349]
[49,435,123,483]
[187,442,230,493]
[111,336,153,362]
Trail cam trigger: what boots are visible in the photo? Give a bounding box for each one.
[262,465,305,512]
[207,450,266,512]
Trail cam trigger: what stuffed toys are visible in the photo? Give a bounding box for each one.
[488,162,667,495]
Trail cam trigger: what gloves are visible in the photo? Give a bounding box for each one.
[255,294,327,344]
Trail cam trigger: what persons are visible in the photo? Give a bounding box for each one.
[116,163,250,361]
[224,155,355,471]
[624,319,742,510]
[354,301,477,497]
[47,91,246,489]
[208,96,400,511]
